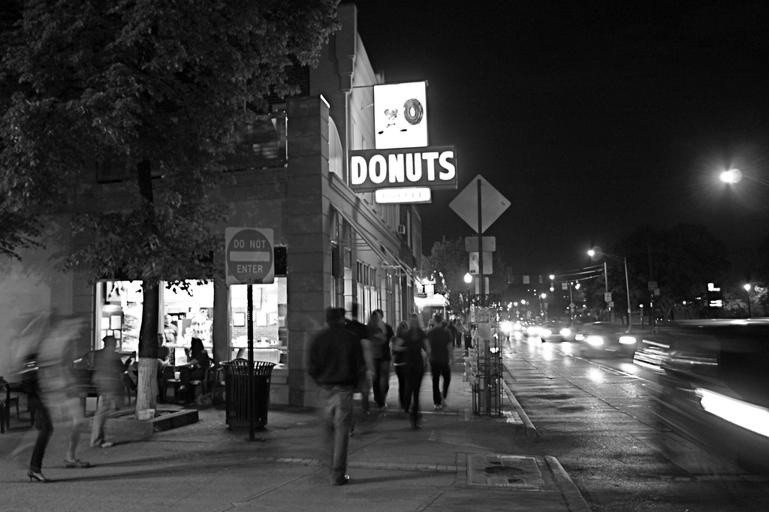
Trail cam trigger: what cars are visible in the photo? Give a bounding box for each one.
[523,321,575,343]
[580,321,637,358]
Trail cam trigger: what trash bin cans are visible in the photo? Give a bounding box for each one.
[219,360,277,430]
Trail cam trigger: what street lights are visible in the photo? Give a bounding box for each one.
[464,273,473,315]
[587,246,632,334]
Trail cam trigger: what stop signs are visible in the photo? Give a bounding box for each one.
[225,227,275,284]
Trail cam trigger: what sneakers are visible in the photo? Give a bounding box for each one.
[98,440,114,448]
[331,474,349,486]
[64,459,89,468]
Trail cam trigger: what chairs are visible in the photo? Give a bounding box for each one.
[168,366,205,405]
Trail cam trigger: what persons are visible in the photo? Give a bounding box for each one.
[400,310,424,421]
[345,301,376,438]
[419,313,466,347]
[365,308,393,411]
[175,335,209,381]
[425,312,451,410]
[158,332,170,370]
[20,344,52,482]
[86,333,129,449]
[307,304,364,486]
[391,319,411,412]
[58,331,91,468]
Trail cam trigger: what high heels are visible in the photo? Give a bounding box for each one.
[27,470,50,483]
